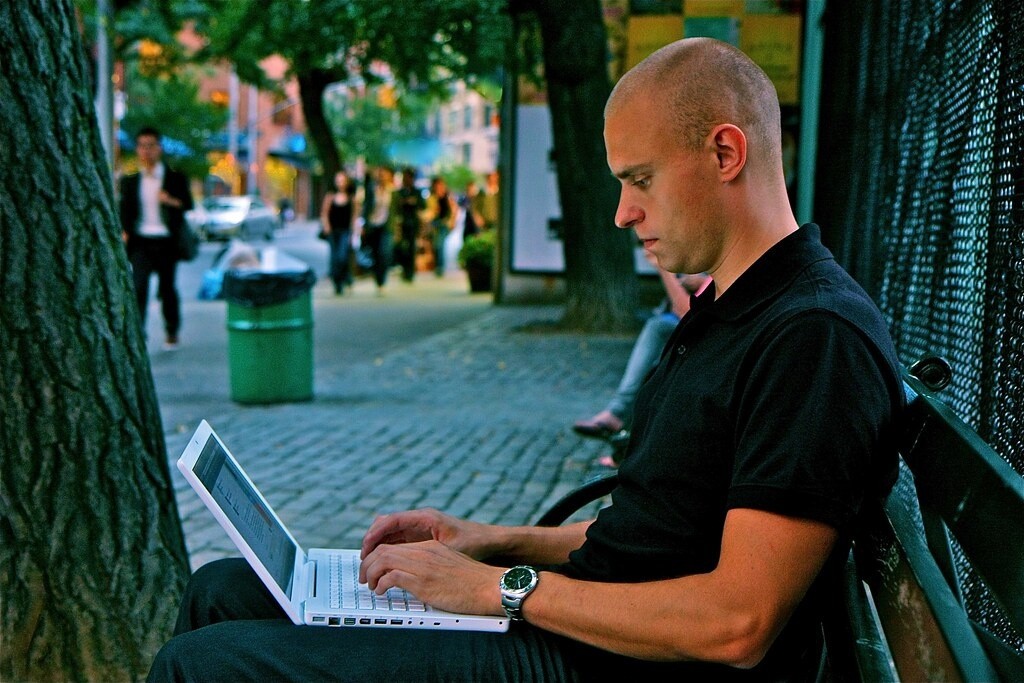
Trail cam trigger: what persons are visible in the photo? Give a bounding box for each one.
[118,127,195,352]
[322,165,498,298]
[574,245,707,472]
[145,37,908,683]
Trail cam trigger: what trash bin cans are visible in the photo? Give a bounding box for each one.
[226,267,314,404]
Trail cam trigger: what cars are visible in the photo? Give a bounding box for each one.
[194,195,275,242]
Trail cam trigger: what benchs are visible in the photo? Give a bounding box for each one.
[793,349,1024,683]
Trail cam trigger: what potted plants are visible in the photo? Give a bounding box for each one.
[456,230,496,293]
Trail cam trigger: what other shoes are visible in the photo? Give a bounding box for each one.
[163,342,178,352]
[599,456,619,468]
[573,411,623,438]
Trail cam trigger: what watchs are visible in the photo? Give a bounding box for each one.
[499,566,540,623]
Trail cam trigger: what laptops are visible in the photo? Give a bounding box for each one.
[177,418,509,633]
